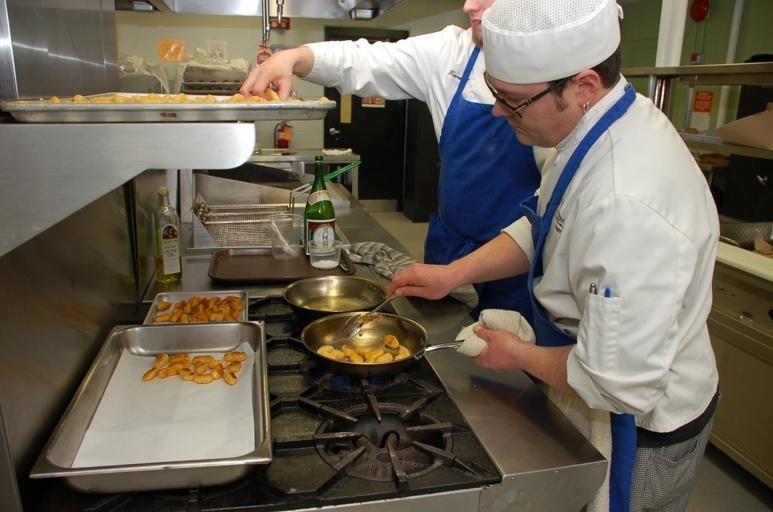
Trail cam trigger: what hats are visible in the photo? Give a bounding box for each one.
[481,0,624,86]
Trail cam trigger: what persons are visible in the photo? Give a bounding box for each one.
[236,0,532,342]
[384,0,719,511]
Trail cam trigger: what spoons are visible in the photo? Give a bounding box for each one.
[342,292,398,338]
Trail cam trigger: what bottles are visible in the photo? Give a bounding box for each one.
[149,184,183,284]
[303,160,335,254]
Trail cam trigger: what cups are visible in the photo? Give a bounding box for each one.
[267,214,301,263]
[305,237,343,271]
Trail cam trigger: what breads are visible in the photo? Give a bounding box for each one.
[142,352,246,385]
[153,295,245,323]
[316,335,411,364]
[17,86,330,104]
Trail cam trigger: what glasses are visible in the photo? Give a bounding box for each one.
[483,70,572,118]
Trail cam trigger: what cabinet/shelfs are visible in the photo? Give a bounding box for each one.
[620,61,773,490]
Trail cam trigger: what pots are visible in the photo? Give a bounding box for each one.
[300,311,464,377]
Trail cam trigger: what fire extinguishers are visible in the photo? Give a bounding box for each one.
[274,122,293,148]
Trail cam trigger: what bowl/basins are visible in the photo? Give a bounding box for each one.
[280,275,385,320]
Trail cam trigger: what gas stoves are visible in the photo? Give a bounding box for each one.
[17,294,505,512]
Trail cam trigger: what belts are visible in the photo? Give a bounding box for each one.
[635,384,720,448]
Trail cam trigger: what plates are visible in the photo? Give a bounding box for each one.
[180,80,242,95]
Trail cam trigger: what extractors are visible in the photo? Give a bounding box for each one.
[2,2,260,258]
